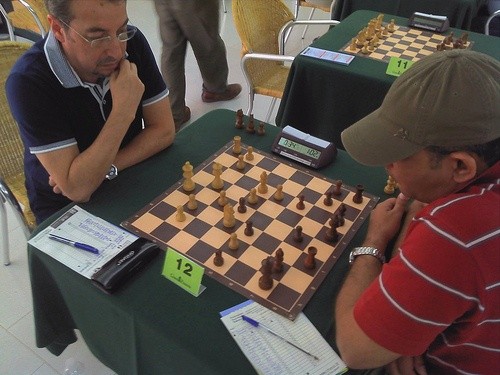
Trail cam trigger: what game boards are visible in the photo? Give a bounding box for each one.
[120,139,381,321]
[338,21,475,63]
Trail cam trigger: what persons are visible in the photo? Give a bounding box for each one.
[4,0,176,226]
[333,49,500,375]
[153,0,242,133]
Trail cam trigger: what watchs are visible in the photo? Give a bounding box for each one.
[348,247,386,266]
[105,164,119,181]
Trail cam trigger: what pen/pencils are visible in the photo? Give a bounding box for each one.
[241,314,319,361]
[49,234,98,253]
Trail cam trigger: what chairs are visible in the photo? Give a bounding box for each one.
[232,0,341,124]
[0,0,50,44]
[284,0,330,43]
[0,39,37,266]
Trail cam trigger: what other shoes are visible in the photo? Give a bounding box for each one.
[174,105,191,132]
[202,84,242,102]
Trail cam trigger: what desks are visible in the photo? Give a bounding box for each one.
[275,10,500,150]
[28,108,414,375]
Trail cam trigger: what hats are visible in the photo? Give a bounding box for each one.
[340,48,500,166]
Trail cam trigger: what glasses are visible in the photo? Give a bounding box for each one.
[60,18,138,49]
